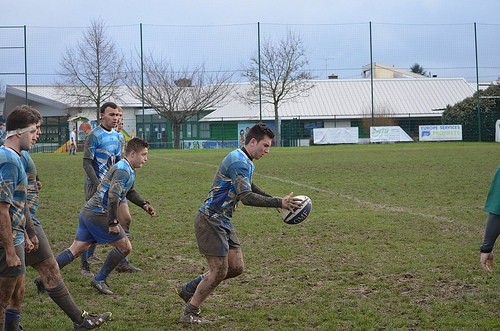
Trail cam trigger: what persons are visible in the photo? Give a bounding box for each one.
[80,102,142,278]
[176,124,304,324]
[114,107,125,163]
[20,108,112,330]
[0,104,35,331]
[35,138,156,295]
[0,115,6,146]
[480,165,500,273]
[70,128,77,156]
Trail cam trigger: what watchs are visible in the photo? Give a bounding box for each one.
[108,219,119,226]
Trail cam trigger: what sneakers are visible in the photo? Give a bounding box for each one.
[178,310,216,325]
[80,260,94,277]
[34,275,45,295]
[115,262,142,273]
[177,283,201,316]
[86,255,104,264]
[73,309,112,331]
[90,276,113,295]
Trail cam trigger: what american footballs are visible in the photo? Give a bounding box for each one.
[281,195,312,225]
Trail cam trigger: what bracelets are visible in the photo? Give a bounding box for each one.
[142,202,149,207]
